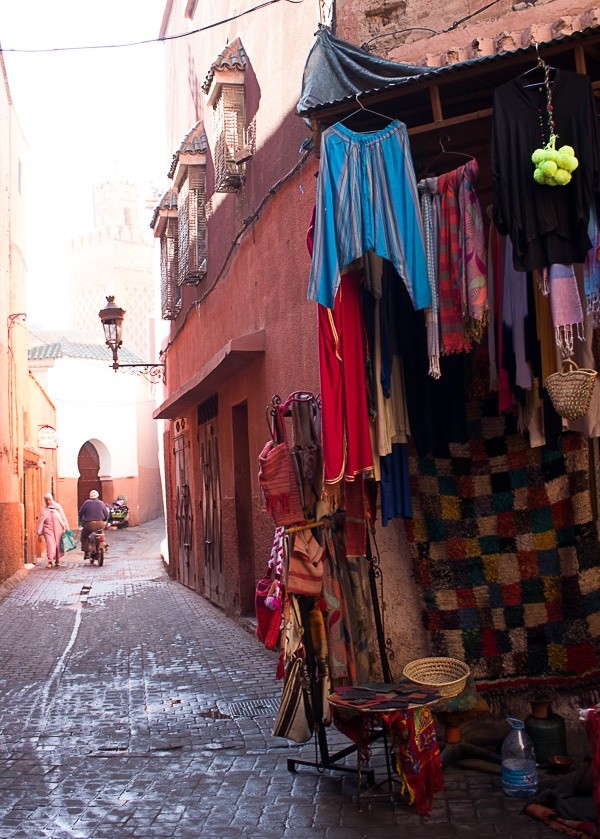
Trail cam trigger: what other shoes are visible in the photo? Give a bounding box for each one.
[46,562,53,568]
[55,561,61,566]
[84,552,90,559]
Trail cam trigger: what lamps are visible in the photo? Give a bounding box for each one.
[98,295,167,385]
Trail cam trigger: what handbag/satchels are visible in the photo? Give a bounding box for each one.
[60,529,77,553]
[256,404,331,741]
[546,357,598,419]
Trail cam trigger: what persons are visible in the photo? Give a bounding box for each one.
[79,490,110,560]
[36,493,69,568]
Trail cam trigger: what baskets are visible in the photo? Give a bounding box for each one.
[402,657,469,698]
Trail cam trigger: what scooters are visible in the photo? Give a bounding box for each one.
[81,519,107,566]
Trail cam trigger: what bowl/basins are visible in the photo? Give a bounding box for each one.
[547,756,571,775]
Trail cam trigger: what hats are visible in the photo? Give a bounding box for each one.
[90,490,99,498]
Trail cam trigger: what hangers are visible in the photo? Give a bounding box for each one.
[413,135,481,191]
[322,86,406,147]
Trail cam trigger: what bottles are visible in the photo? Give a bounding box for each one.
[500,716,542,798]
